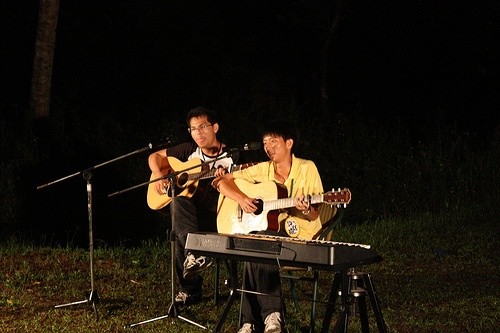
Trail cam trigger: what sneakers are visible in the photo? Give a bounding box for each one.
[168,291,202,315]
[264,311,282,333]
[182,252,212,279]
[237,322,257,333]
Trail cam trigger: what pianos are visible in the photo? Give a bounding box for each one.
[184,231,388,333]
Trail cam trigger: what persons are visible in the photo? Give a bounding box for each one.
[148,105,240,317]
[210,120,325,333]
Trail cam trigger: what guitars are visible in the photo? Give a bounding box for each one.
[146,156,263,216]
[216,177,352,235]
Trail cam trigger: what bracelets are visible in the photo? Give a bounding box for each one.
[301,209,311,216]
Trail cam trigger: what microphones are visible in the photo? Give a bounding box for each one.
[144,137,179,153]
[223,143,264,152]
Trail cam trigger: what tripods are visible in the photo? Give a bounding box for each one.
[36,147,145,323]
[108,152,241,332]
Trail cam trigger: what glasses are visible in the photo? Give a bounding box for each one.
[189,123,214,133]
